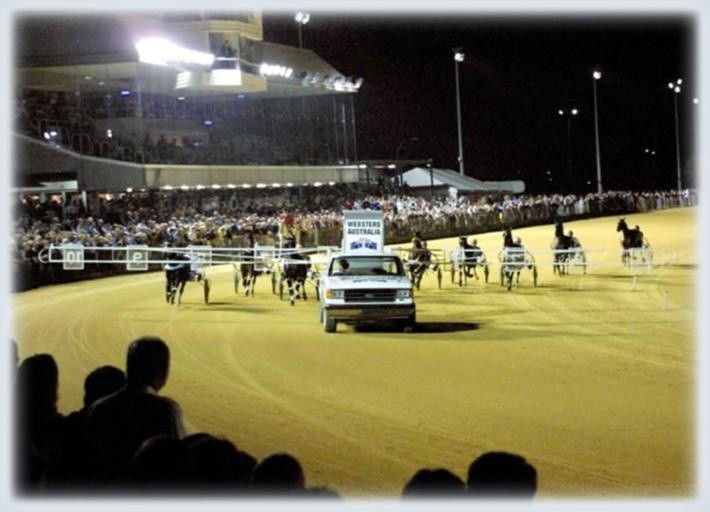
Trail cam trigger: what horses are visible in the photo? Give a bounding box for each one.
[407,236,431,290]
[458,235,479,281]
[162,238,190,307]
[240,228,257,298]
[285,234,308,305]
[615,217,645,262]
[552,218,586,276]
[501,224,521,291]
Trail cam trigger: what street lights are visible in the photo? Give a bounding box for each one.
[558,108,582,140]
[295,12,312,48]
[590,71,605,193]
[666,78,685,190]
[132,33,364,94]
[452,51,467,175]
[396,136,419,189]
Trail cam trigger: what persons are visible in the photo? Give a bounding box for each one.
[14,332,537,498]
[14,42,694,279]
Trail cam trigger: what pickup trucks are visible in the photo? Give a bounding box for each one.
[317,249,416,335]
